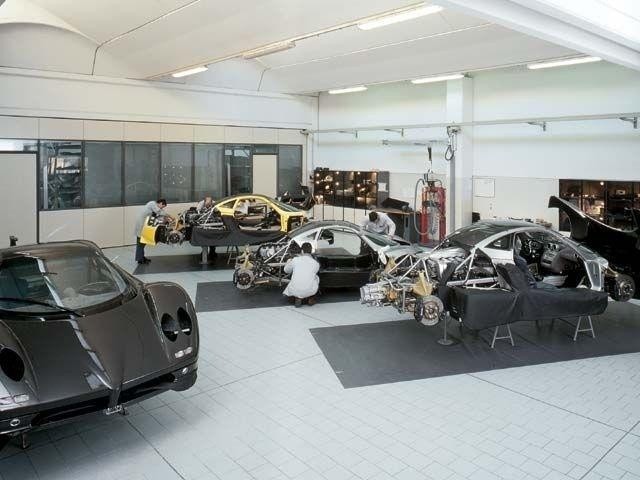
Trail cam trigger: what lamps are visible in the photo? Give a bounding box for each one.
[242,44,293,61]
[360,4,446,31]
[328,86,368,95]
[411,72,466,85]
[172,66,209,78]
[527,57,603,71]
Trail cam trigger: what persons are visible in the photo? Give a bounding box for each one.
[281,242,321,306]
[359,210,397,242]
[133,198,168,265]
[195,196,218,258]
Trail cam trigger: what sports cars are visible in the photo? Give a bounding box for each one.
[0,235,200,449]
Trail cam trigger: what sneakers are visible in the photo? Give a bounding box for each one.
[138,257,151,265]
[306,296,321,306]
[294,298,302,307]
